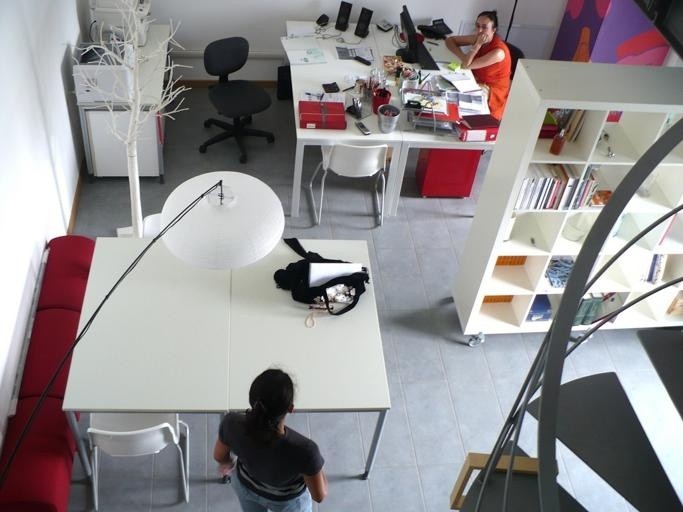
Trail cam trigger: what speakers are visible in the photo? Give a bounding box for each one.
[354,7,373,38]
[335,1,352,32]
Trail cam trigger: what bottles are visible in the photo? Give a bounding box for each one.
[549,128,566,156]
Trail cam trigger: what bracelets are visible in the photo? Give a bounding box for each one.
[469,49,477,54]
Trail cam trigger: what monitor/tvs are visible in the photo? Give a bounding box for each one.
[396,5,419,63]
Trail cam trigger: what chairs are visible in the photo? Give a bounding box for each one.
[306,142,389,226]
[503,40,526,80]
[198,35,274,164]
[144,212,162,238]
[86,411,191,512]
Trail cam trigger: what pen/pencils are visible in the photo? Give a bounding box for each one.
[362,76,385,96]
[355,56,370,65]
[343,86,355,92]
[426,40,439,46]
[305,92,317,96]
[436,60,450,63]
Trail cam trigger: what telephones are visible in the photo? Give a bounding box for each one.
[376,21,392,32]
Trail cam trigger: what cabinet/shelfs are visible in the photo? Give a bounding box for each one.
[451,58,683,346]
[75,24,172,184]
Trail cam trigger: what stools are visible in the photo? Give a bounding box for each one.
[0,233,95,512]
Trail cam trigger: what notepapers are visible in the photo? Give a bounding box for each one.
[448,60,462,71]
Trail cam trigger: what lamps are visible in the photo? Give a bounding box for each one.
[0,171,286,512]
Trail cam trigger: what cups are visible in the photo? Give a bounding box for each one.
[377,104,401,134]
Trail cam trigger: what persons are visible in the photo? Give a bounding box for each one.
[444,10,513,121]
[212,366,327,510]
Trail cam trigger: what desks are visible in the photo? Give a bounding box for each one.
[62,238,392,479]
[286,24,403,220]
[370,23,497,218]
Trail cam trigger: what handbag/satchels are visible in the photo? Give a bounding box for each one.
[274,257,369,304]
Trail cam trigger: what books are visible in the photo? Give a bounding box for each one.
[306,261,362,305]
[526,255,682,326]
[510,163,616,211]
[278,34,327,68]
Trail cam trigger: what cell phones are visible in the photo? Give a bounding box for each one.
[356,122,371,134]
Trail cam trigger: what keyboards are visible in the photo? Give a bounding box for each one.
[416,41,440,70]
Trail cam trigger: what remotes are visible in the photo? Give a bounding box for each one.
[353,56,371,65]
